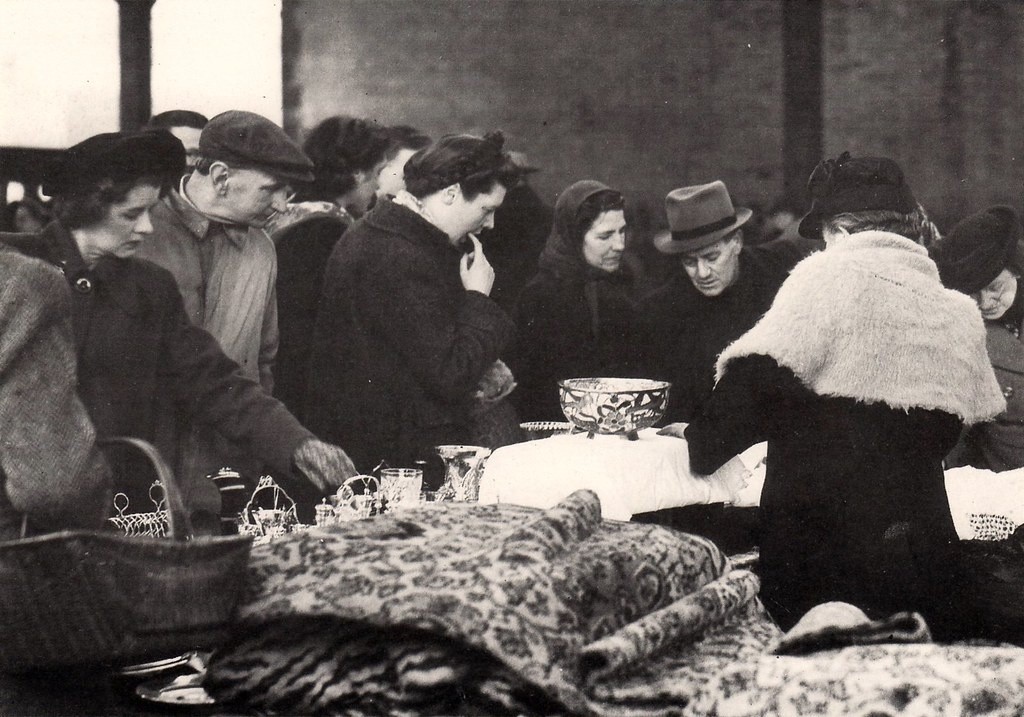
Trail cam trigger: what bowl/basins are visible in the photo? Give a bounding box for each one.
[557,372,671,439]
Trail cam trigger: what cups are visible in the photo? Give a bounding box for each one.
[380,468,423,512]
[257,509,284,538]
[434,444,492,498]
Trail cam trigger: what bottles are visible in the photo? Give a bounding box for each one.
[314,483,374,527]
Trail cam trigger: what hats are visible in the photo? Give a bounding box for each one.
[199,110,316,183]
[497,151,542,177]
[928,203,1022,295]
[799,151,917,239]
[43,129,187,204]
[652,180,753,254]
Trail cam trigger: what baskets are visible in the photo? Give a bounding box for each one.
[0,433,252,686]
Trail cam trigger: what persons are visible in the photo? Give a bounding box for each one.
[513,180,645,431]
[0,110,1024,716]
